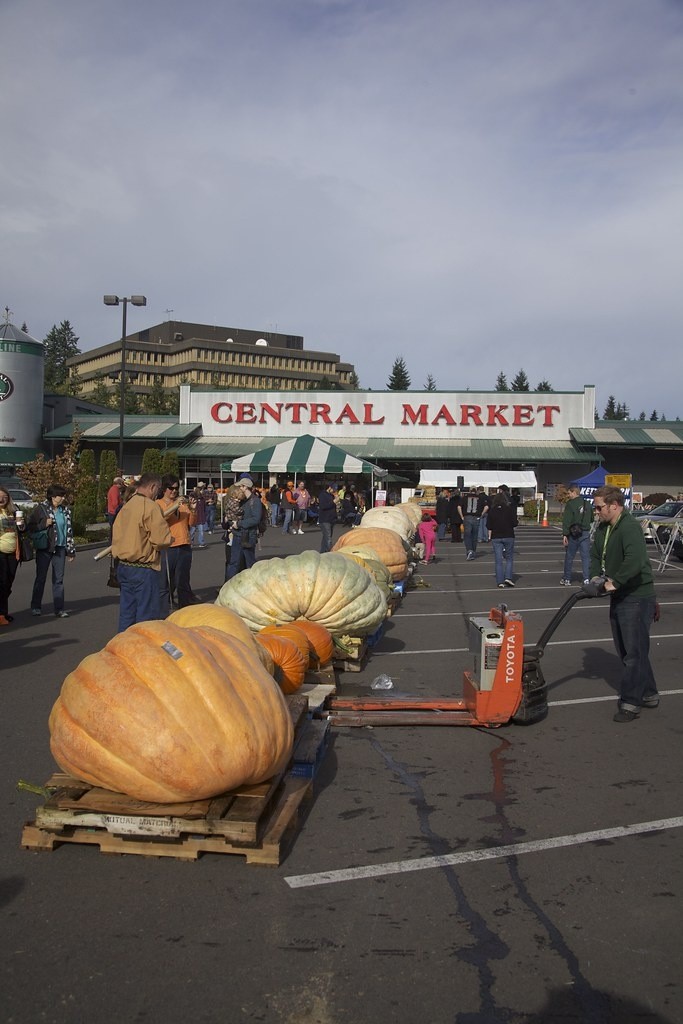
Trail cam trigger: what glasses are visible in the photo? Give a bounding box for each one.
[167,487,179,491]
[593,504,608,512]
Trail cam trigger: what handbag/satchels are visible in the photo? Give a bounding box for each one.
[570,524,582,538]
[107,567,122,589]
[20,539,33,561]
[30,530,48,549]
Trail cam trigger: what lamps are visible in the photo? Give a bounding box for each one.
[173,332,183,341]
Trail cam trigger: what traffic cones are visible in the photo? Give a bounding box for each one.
[541,511,550,527]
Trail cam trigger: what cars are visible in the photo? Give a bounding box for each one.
[8,488,39,513]
[634,499,683,543]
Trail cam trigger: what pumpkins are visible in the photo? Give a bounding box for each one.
[48,503,424,801]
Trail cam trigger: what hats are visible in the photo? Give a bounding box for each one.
[234,478,253,488]
[287,482,293,486]
[470,485,477,491]
[197,482,205,486]
[240,472,253,480]
[208,484,213,487]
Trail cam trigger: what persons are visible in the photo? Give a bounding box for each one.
[487,484,520,588]
[319,482,340,553]
[435,486,497,544]
[589,485,660,722]
[221,473,267,583]
[107,472,217,632]
[269,481,319,534]
[334,484,370,528]
[28,484,76,618]
[457,485,489,561]
[419,512,438,566]
[558,486,594,585]
[0,486,26,626]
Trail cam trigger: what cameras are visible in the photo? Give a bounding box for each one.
[183,499,190,504]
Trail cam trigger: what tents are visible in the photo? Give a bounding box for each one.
[420,471,537,499]
[570,466,631,509]
[220,434,389,508]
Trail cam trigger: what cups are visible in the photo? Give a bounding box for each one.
[15,511,24,526]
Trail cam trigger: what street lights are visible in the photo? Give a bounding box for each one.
[102,294,147,478]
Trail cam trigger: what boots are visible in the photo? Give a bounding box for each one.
[241,535,252,548]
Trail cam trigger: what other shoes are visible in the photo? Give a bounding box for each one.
[613,708,635,722]
[643,698,660,708]
[199,544,207,548]
[57,611,68,617]
[559,579,571,586]
[418,560,427,565]
[466,552,475,560]
[431,555,436,560]
[32,609,41,616]
[583,579,590,585]
[499,579,515,587]
[0,616,13,625]
[298,529,304,534]
